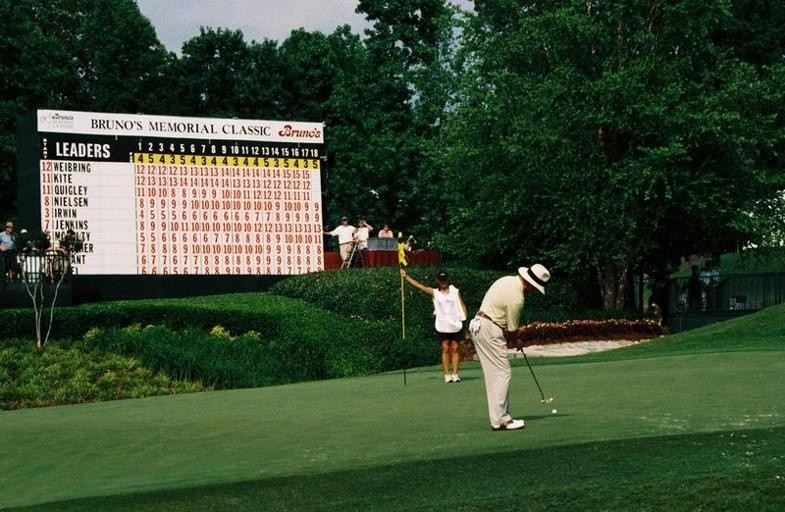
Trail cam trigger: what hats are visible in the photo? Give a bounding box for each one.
[4,221,14,228]
[340,217,348,221]
[518,263,551,295]
[437,271,449,281]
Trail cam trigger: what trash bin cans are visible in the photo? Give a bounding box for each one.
[366,237,399,250]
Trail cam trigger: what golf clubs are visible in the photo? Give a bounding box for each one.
[519,347,553,404]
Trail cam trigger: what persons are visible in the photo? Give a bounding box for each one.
[399,268,468,383]
[323,216,357,270]
[404,235,417,265]
[468,262,551,431]
[352,217,374,268]
[0,222,19,279]
[378,224,394,239]
[397,232,409,267]
[55,228,76,274]
[20,229,39,252]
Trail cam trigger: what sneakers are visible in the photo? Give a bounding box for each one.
[491,418,525,430]
[444,373,461,383]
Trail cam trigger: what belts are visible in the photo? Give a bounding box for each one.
[339,241,352,245]
[477,311,505,330]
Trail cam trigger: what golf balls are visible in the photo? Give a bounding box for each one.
[551,408,558,414]
